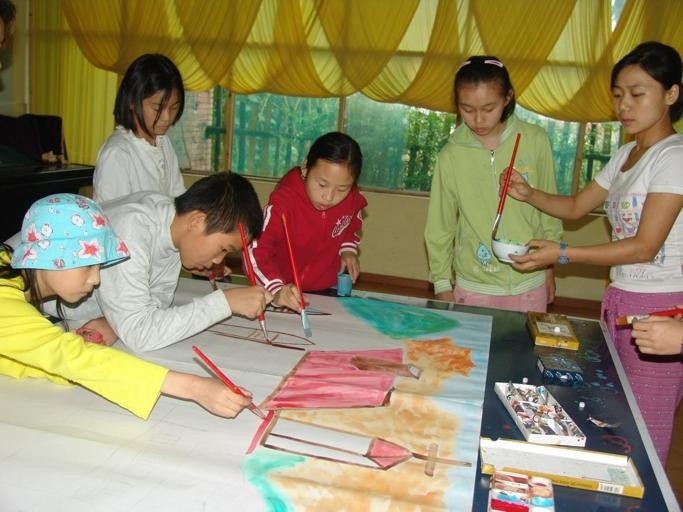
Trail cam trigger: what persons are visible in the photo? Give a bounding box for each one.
[242,133,368,312]
[0,172,274,350]
[92,52,232,280]
[499,40,682,470]
[630,304,682,355]
[425,55,564,312]
[0,194,253,420]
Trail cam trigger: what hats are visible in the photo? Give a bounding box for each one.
[10,193,131,271]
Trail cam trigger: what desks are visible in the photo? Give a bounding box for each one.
[0,277,681,512]
[0,162,95,245]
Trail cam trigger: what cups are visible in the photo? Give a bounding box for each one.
[337,273,352,296]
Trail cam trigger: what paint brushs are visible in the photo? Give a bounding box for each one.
[615,308,683,326]
[492,133,521,239]
[208,273,218,291]
[282,212,312,338]
[191,345,271,422]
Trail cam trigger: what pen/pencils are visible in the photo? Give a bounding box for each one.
[238,222,272,344]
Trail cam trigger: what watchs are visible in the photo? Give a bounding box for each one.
[559,243,569,267]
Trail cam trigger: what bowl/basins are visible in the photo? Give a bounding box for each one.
[491,239,530,264]
